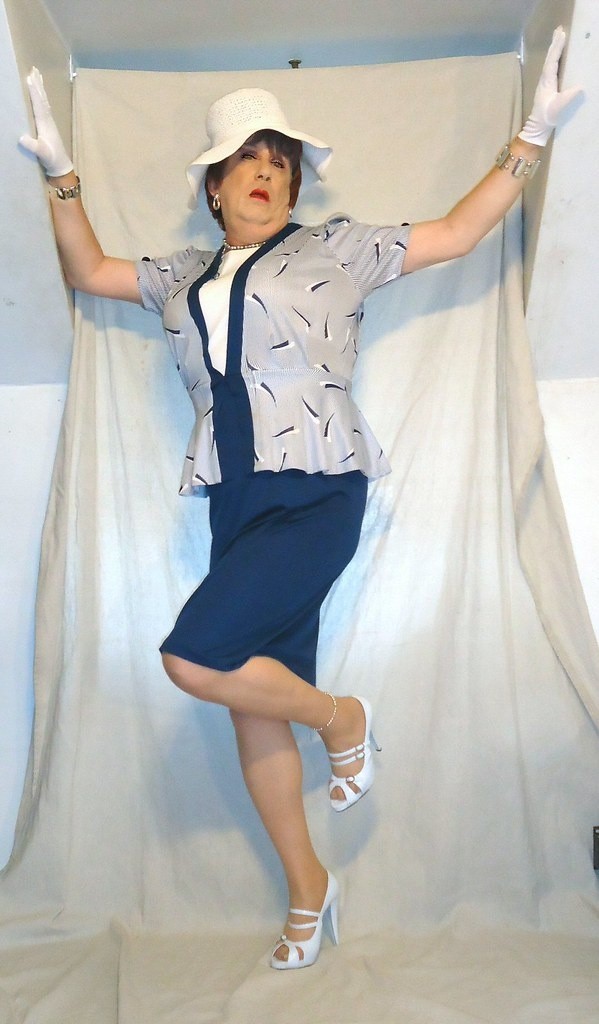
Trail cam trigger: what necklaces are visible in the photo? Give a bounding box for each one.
[222,238,270,250]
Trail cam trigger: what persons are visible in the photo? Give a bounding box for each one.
[14,23,587,973]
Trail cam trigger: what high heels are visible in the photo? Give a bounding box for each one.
[328,697,383,811]
[270,871,341,968]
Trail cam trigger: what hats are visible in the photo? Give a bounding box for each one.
[185,88,332,210]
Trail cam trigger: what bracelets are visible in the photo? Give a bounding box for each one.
[48,175,82,200]
[495,145,541,180]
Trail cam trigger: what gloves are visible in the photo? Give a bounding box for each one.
[18,66,75,177]
[518,26,585,147]
